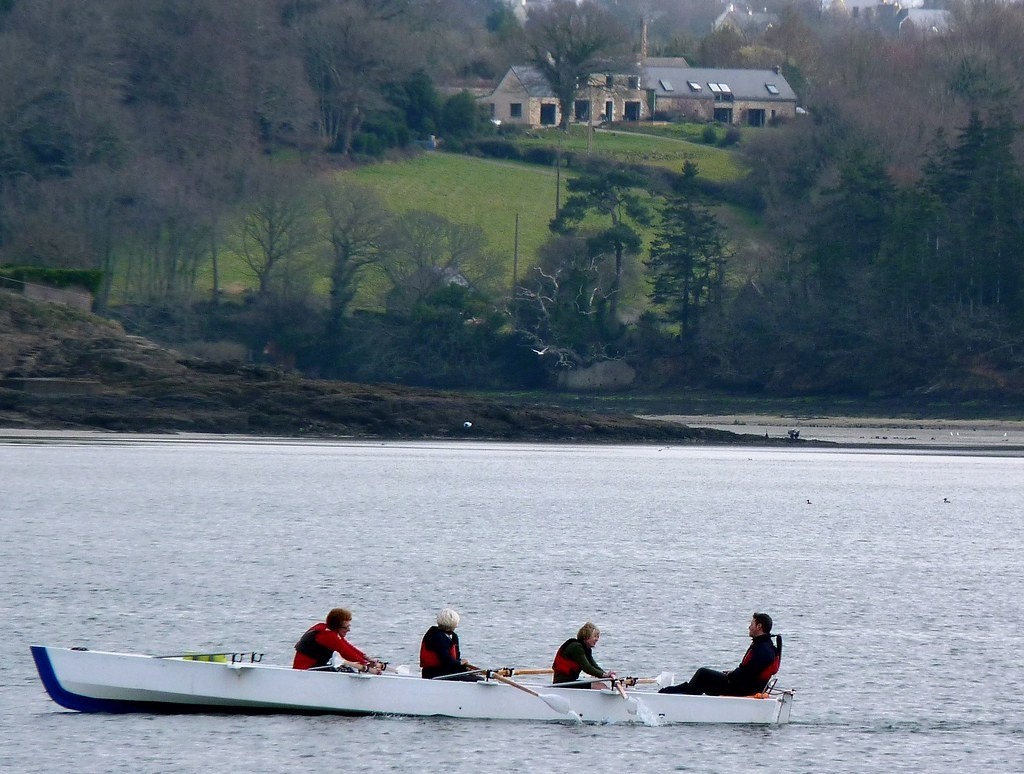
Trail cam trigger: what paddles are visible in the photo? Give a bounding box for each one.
[477,668,554,677]
[609,674,639,717]
[372,660,410,676]
[330,651,378,675]
[467,664,571,715]
[616,670,676,688]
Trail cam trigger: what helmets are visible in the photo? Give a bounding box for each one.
[437,608,460,628]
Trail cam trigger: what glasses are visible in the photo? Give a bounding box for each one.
[339,624,350,629]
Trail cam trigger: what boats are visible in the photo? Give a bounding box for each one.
[31,646,793,725]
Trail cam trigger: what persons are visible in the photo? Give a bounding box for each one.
[684,613,777,697]
[419,608,485,682]
[552,622,616,689]
[292,608,380,675]
